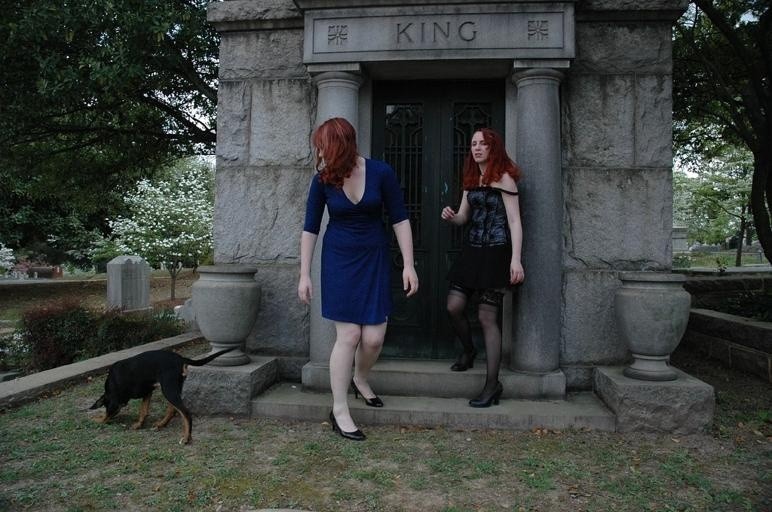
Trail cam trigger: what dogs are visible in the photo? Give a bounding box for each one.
[88,346,246,445]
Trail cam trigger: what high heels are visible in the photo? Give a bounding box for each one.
[351,376,383,408]
[469,381,504,408]
[330,409,366,440]
[451,347,478,371]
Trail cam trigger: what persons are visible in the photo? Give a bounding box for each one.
[440,124,525,406]
[297,117,419,440]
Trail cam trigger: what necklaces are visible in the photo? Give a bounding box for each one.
[479,172,485,187]
